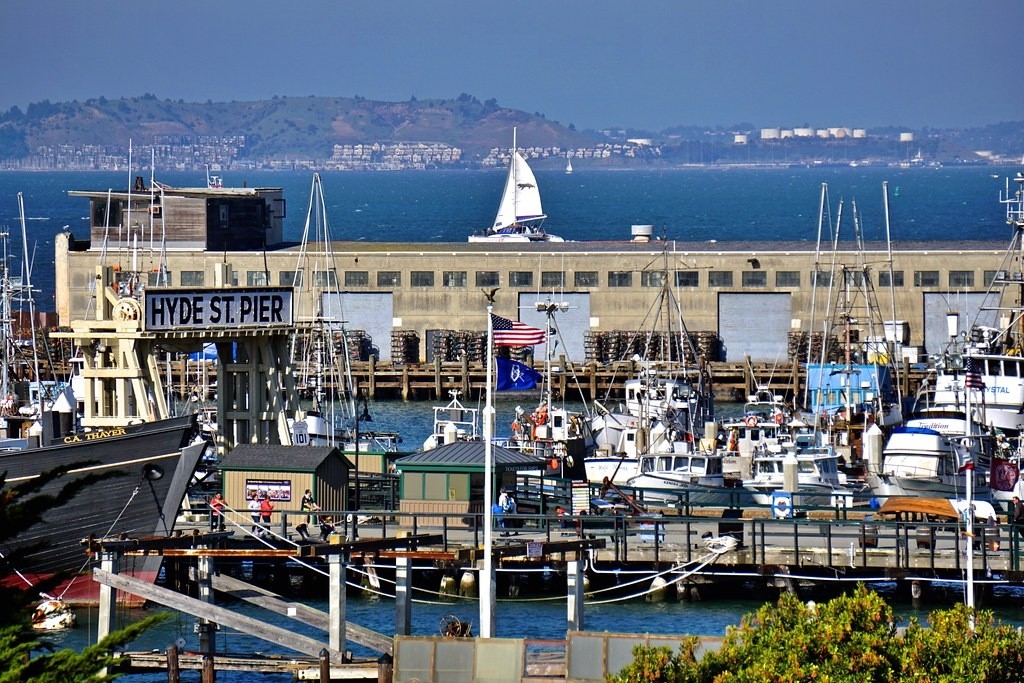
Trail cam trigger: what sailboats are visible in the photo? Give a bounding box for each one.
[465,126,567,242]
[0,137,1024,525]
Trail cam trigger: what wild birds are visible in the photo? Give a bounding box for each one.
[481,288,501,304]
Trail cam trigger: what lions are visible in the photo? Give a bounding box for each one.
[518,183,536,190]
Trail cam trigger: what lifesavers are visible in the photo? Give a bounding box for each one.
[774,497,791,516]
[745,415,758,427]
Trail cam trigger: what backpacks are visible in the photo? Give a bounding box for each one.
[503,495,515,514]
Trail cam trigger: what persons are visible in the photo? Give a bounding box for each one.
[1012,496,1024,539]
[498,487,518,537]
[300,489,335,543]
[555,506,570,538]
[210,492,228,533]
[247,494,275,536]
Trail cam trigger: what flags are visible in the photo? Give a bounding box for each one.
[491,314,547,345]
[496,355,543,392]
